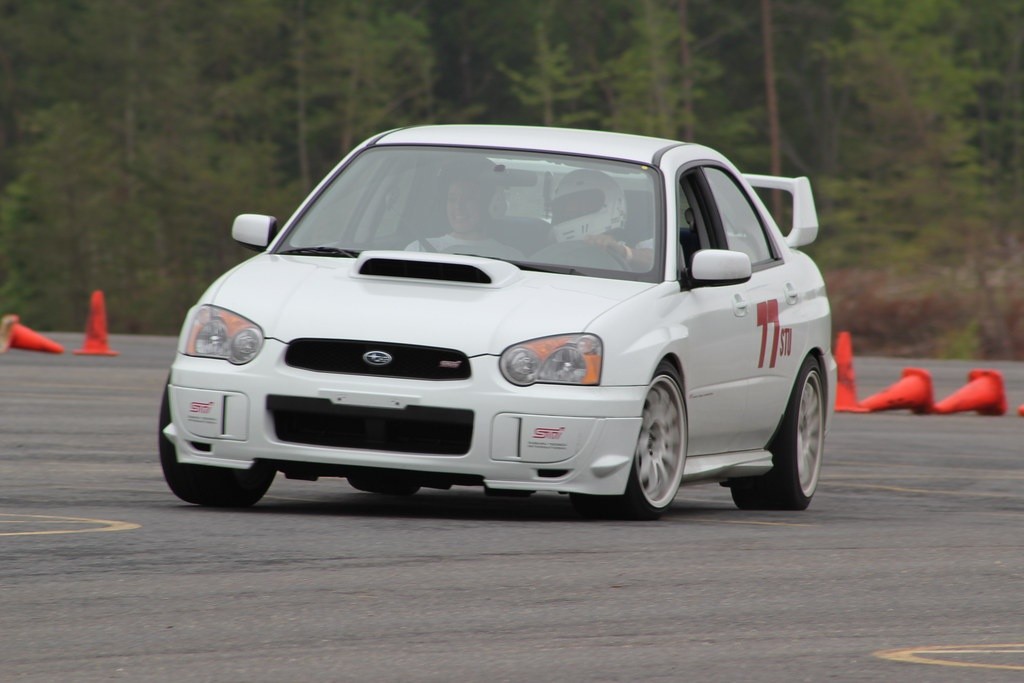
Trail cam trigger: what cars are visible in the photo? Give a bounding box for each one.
[157,122,839,523]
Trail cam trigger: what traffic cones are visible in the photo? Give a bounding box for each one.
[859,367,934,416]
[1,314,64,354]
[73,289,119,357]
[830,331,866,412]
[932,369,1008,416]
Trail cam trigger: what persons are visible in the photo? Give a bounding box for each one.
[531,169,681,271]
[408,171,526,260]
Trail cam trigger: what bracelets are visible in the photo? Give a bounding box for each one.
[622,243,634,260]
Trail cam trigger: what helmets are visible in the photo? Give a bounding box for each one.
[549,168,627,244]
